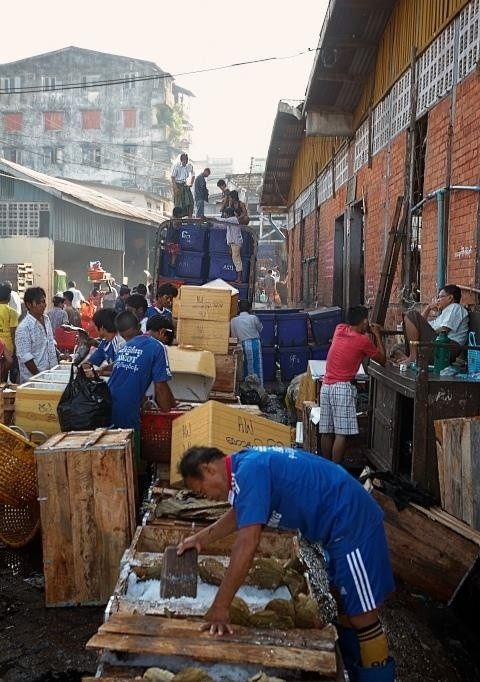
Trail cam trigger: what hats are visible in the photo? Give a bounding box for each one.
[54,292,65,299]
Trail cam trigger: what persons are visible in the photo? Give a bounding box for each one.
[172,444,396,682]
[171,153,195,223]
[311,305,389,465]
[256,267,283,309]
[0,274,178,472]
[210,207,244,284]
[392,284,472,377]
[194,167,211,218]
[229,299,266,388]
[164,206,185,269]
[227,189,249,225]
[216,179,231,218]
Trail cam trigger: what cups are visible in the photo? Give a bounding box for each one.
[400,364,408,372]
[396,325,403,331]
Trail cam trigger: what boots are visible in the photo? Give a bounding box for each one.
[331,614,395,682]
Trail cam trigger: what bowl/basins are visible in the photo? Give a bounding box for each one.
[87,271,105,280]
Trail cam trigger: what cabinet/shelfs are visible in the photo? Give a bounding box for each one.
[362,358,480,504]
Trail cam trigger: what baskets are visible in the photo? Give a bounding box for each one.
[1,424,40,549]
[141,409,186,463]
[468,332,480,373]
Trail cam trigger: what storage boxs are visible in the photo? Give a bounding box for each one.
[433,416,480,531]
[357,466,480,612]
